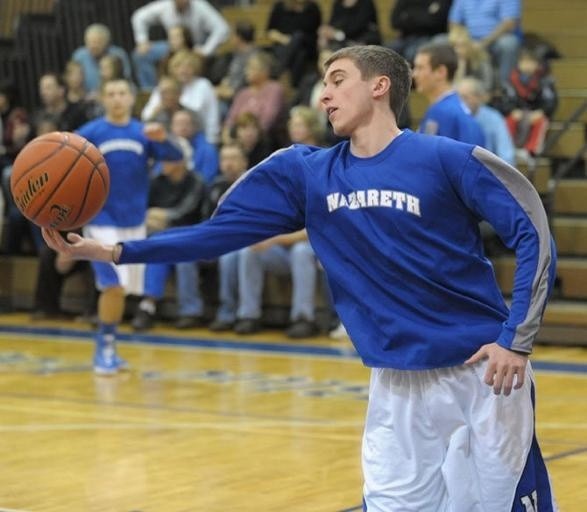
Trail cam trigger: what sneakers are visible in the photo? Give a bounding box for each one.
[94,335,127,375]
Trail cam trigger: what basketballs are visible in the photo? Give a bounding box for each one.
[10,131,110,231]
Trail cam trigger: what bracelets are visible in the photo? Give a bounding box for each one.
[334,30,346,41]
[112,246,117,265]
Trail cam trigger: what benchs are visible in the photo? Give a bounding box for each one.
[0,0,586,347]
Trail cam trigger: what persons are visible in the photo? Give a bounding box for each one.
[0,0,558,337]
[69,77,187,375]
[40,45,559,512]
